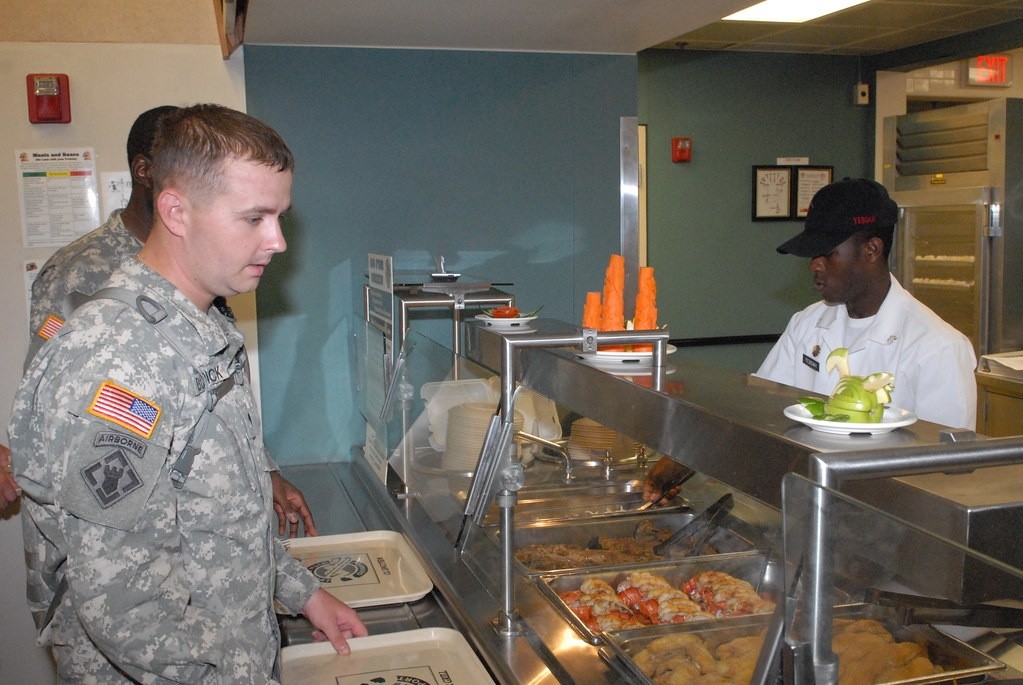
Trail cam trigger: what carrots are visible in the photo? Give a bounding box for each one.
[583,254,658,353]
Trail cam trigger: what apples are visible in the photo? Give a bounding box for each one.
[796,348,894,423]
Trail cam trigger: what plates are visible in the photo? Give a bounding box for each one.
[567,417,637,460]
[442,403,524,470]
[784,404,918,435]
[275,530,433,616]
[280,627,497,685]
[563,343,677,362]
[474,314,538,328]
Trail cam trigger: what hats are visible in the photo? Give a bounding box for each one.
[777,177,899,259]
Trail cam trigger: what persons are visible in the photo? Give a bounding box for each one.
[641,176,976,586]
[10,103,368,684]
[22,106,320,537]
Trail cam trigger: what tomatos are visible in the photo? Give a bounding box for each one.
[492,305,518,318]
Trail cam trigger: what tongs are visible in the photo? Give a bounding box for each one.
[633,469,697,509]
[863,587,1023,630]
[654,491,734,558]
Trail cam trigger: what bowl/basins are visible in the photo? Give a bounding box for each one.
[421,376,563,452]
